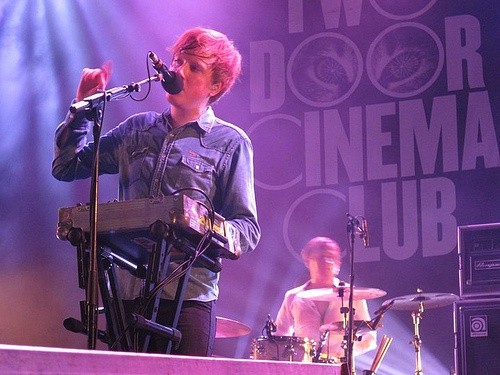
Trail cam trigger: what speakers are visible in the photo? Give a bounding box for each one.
[453,298,500,375]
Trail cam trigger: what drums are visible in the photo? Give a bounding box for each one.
[251,335,312,363]
[311,354,338,365]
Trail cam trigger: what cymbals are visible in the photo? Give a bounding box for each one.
[215,315,253,339]
[295,286,388,302]
[319,320,384,332]
[382,293,461,311]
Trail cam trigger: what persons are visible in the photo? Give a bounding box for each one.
[271,236,377,361]
[51,28,261,356]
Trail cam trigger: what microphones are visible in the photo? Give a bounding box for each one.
[362,217,370,248]
[149,52,183,94]
[373,309,386,330]
[268,316,277,332]
[318,330,329,347]
